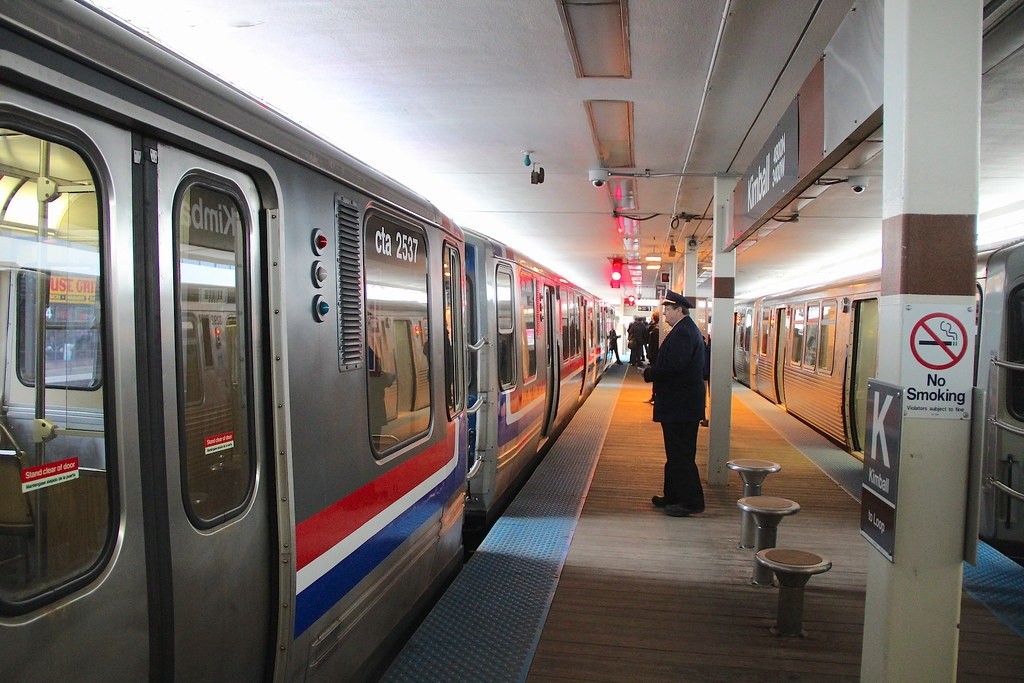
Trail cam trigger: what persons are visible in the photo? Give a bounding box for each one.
[643,308,660,404]
[364,344,397,459]
[641,288,708,516]
[608,315,651,367]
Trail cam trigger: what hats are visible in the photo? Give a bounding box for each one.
[660,289,694,308]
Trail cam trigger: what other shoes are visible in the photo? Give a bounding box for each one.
[652,494,674,507]
[665,505,690,517]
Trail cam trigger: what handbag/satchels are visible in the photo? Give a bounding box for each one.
[628,338,638,349]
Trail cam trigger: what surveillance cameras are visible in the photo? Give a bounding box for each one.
[848,176,869,193]
[589,170,608,187]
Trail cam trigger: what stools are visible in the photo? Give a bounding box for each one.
[736,495,802,590]
[726,458,782,551]
[756,548,832,639]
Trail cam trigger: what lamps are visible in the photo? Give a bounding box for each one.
[628,295,635,303]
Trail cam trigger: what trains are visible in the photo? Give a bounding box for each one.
[708,236,1024,569]
[0,0,623,683]
